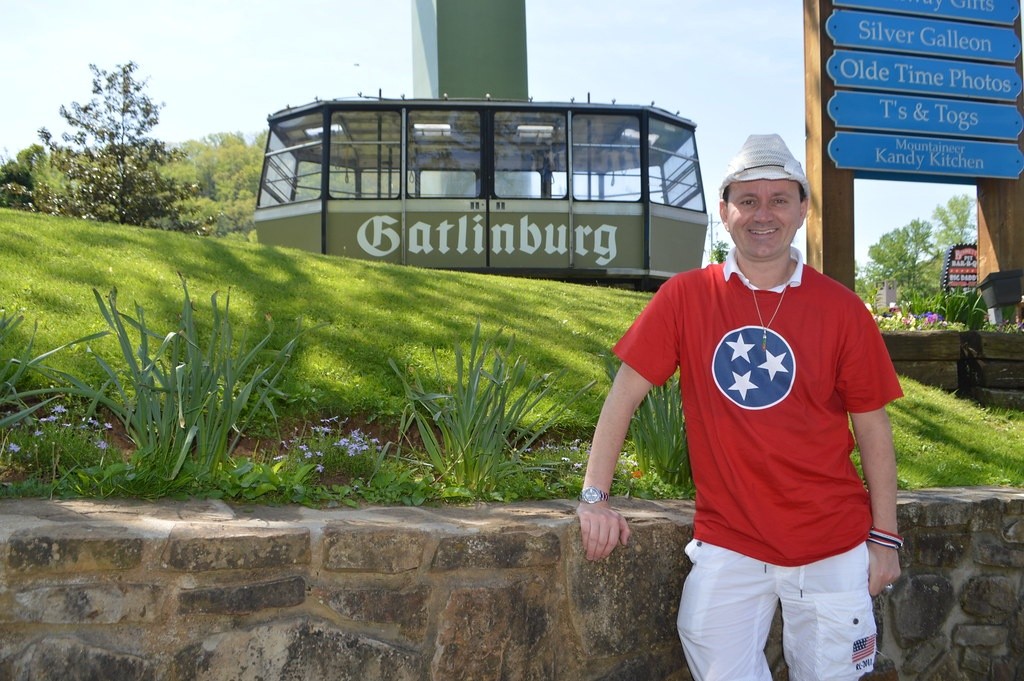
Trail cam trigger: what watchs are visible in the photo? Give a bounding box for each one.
[581,486,608,504]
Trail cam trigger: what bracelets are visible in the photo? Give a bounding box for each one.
[867,528,904,548]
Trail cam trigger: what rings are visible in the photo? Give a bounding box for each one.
[885,584,893,590]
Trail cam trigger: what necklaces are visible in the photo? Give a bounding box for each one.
[743,261,795,350]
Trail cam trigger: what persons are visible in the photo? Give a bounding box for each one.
[578,133,903,681]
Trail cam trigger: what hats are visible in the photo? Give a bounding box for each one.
[889,302,899,313]
[719,132,810,199]
